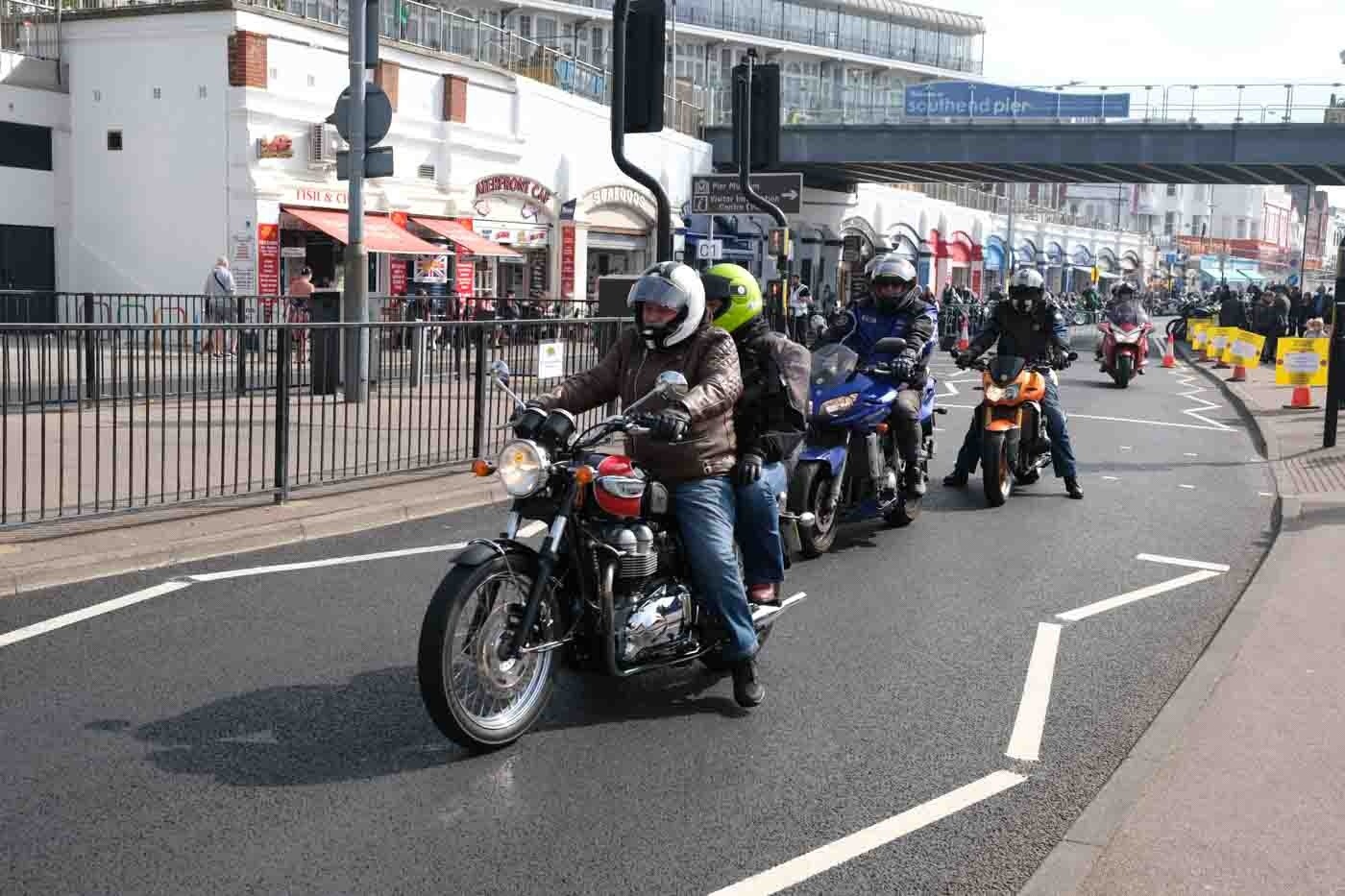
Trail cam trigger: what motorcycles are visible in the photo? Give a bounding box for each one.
[1095,301,1156,389]
[416,358,818,753]
[786,312,949,557]
[936,290,1102,348]
[1153,292,1223,338]
[951,346,1077,506]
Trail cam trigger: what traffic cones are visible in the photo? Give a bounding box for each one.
[959,318,969,350]
[1161,332,1176,367]
[1226,366,1260,382]
[1283,384,1321,409]
[1195,350,1230,369]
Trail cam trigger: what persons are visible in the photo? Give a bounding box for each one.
[391,288,555,350]
[200,255,239,358]
[1082,280,1154,376]
[288,266,316,364]
[509,261,767,709]
[1178,282,1335,364]
[787,255,1009,496]
[698,264,812,605]
[942,269,1085,498]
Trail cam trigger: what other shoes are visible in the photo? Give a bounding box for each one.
[904,460,927,497]
[942,467,969,485]
[1064,477,1084,499]
[1138,369,1146,376]
[747,583,775,604]
[1099,363,1105,373]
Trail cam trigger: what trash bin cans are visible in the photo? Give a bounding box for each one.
[311,291,383,394]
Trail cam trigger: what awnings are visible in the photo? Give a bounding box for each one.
[279,205,524,258]
[1074,266,1120,278]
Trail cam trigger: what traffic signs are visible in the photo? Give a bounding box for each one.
[691,173,804,214]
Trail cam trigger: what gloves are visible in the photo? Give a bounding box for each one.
[508,399,544,438]
[737,453,762,486]
[650,406,692,442]
[1052,346,1071,372]
[954,350,976,370]
[890,355,917,379]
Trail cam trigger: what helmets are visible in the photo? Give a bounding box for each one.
[864,254,917,312]
[699,263,763,337]
[626,261,706,350]
[1008,268,1045,314]
[1110,280,1135,300]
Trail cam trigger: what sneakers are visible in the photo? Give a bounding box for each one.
[732,659,766,709]
